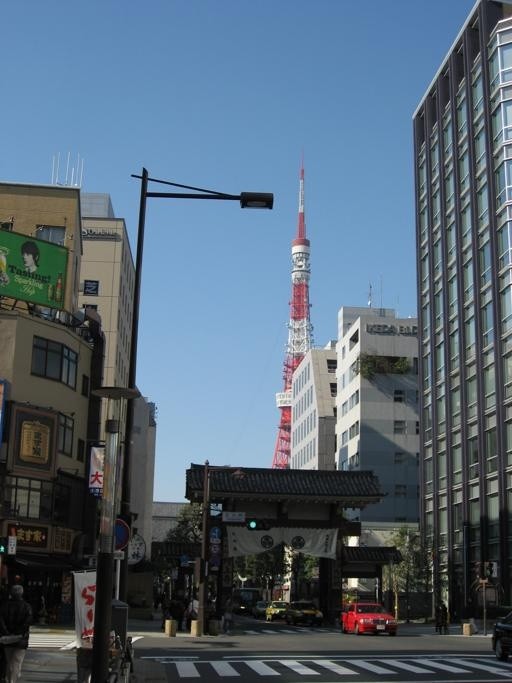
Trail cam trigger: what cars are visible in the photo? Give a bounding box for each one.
[339,601,398,636]
[285,600,324,626]
[266,600,289,620]
[490,610,512,661]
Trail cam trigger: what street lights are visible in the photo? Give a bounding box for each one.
[108,162,279,653]
[88,385,143,682]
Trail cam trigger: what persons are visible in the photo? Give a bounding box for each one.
[0,578,50,682]
[434,600,450,635]
[153,582,236,634]
[20,241,40,272]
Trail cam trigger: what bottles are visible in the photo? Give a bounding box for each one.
[55,272,64,303]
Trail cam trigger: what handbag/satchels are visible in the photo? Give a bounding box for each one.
[1,636,22,644]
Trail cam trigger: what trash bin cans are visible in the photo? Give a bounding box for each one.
[463,623,472,636]
[165,619,176,637]
[191,619,202,637]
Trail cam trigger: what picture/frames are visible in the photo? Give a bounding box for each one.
[2,400,61,482]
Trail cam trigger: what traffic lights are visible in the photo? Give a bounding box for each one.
[0,536,9,554]
[484,561,493,576]
[246,517,272,530]
[475,561,483,576]
